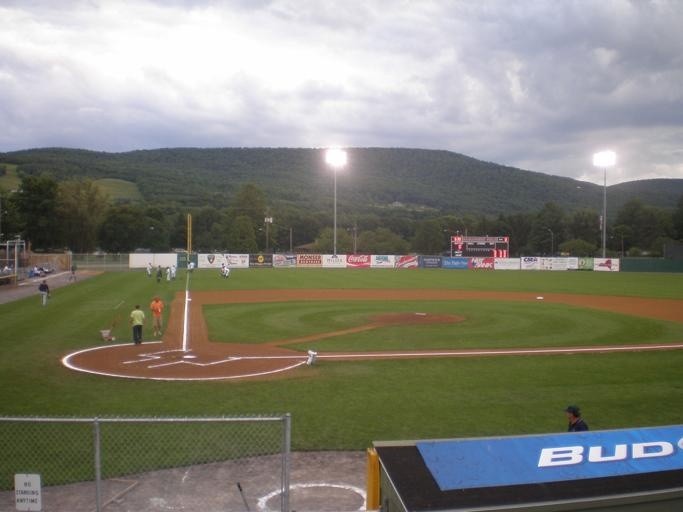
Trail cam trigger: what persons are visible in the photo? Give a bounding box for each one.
[38,279,49,307]
[33,264,43,276]
[150,295,165,336]
[563,406,589,432]
[304,348,318,366]
[129,304,145,345]
[3,263,12,275]
[67,263,79,285]
[38,265,46,274]
[145,259,232,284]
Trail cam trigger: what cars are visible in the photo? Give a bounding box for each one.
[94,250,108,257]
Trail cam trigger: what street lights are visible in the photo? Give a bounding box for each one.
[593,147,616,258]
[546,228,553,258]
[323,147,349,254]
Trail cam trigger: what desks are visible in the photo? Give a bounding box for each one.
[0,275,19,284]
